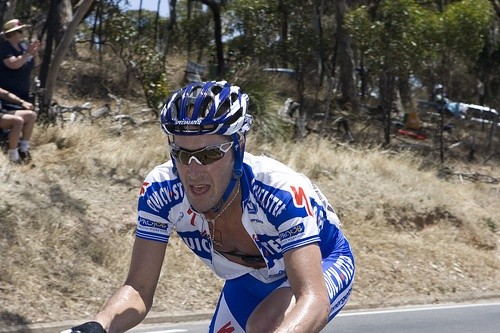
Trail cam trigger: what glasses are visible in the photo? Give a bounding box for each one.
[17,29,23,33]
[171,140,236,164]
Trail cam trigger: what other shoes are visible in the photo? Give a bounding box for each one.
[19,149,32,164]
[10,158,23,166]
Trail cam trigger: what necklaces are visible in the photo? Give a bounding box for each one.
[200,179,240,246]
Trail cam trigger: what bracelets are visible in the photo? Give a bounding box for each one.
[20,100,24,106]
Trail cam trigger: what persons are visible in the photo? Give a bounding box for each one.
[0,19,40,101]
[0,88,37,169]
[61,80,356,333]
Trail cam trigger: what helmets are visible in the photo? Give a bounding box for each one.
[159,79,253,135]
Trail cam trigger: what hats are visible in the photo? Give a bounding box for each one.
[0,18,31,34]
